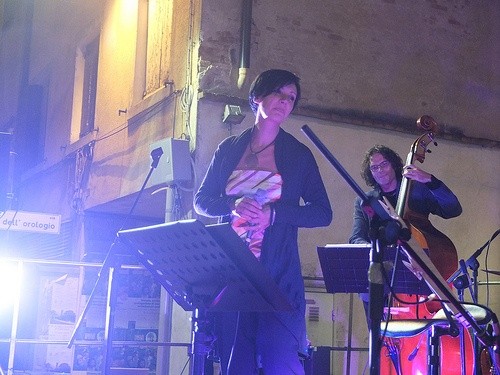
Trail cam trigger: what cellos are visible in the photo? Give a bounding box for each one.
[369,113,492,375]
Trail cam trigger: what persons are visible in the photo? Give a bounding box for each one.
[193,69,333,375]
[348,144,463,316]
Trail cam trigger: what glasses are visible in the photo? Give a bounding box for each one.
[369,160,390,172]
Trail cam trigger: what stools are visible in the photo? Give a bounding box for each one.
[380,302,493,375]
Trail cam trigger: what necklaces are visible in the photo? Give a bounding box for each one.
[244,133,279,168]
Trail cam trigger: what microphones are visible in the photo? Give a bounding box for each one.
[245,189,267,247]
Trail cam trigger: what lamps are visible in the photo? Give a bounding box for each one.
[222,104,246,125]
